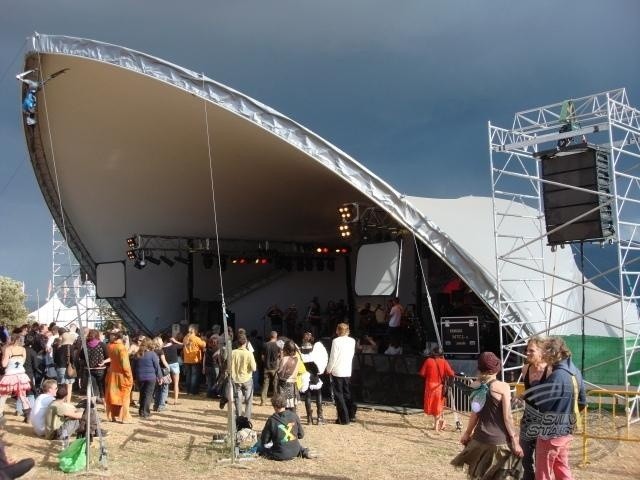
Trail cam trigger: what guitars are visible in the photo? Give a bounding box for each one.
[303,308,312,321]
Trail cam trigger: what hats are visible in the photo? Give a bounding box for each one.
[61,332,76,345]
[478,352,502,374]
[424,343,443,356]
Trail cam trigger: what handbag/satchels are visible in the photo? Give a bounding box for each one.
[491,453,522,480]
[67,363,78,378]
[443,385,452,396]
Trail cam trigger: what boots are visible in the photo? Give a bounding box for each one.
[317,408,327,425]
[306,409,313,425]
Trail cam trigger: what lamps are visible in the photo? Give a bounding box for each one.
[337,203,390,241]
[125,232,354,275]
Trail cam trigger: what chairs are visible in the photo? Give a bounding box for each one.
[348,354,421,407]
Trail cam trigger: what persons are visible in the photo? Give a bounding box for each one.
[0,294,588,480]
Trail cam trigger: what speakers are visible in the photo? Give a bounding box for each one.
[542,150,615,243]
